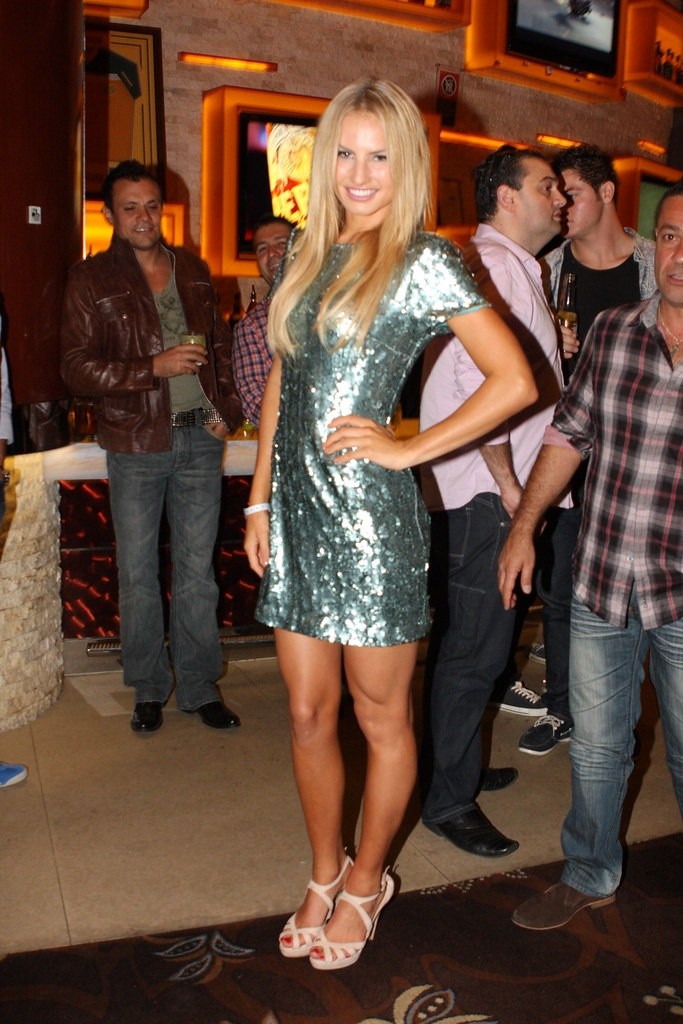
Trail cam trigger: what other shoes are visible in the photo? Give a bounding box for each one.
[0,760,27,787]
[510,873,616,931]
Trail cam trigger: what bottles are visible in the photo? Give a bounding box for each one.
[555,272,578,354]
[228,292,245,331]
[246,284,258,314]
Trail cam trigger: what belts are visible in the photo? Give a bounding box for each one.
[171,408,223,428]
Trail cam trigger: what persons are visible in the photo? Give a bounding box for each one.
[0,344,28,789]
[243,79,543,968]
[238,142,683,930]
[60,162,243,731]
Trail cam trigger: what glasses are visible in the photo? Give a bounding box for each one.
[489,144,518,206]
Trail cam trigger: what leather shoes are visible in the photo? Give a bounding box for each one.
[180,700,242,730]
[478,767,519,791]
[422,804,519,857]
[130,699,164,732]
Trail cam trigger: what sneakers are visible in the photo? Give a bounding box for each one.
[525,641,547,665]
[518,711,573,755]
[497,680,550,716]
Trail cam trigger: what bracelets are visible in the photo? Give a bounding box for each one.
[243,502,271,516]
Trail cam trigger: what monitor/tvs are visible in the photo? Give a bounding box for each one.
[464,0,629,103]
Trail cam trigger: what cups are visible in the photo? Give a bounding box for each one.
[182,335,206,366]
[242,419,256,438]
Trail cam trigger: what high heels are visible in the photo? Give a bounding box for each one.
[278,846,356,958]
[309,864,396,970]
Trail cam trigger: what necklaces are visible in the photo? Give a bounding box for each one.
[659,302,683,358]
